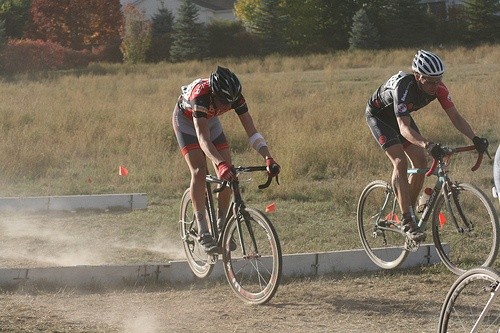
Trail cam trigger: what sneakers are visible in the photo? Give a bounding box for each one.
[196,230,219,254]
[217,224,237,252]
[400,216,427,240]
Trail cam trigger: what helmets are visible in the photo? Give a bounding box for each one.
[209,65,242,103]
[412,48,444,74]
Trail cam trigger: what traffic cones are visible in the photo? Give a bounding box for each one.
[438,213,447,228]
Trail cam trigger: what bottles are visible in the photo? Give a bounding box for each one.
[416,188,432,213]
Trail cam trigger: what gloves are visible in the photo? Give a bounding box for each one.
[217,160,236,181]
[264,157,281,176]
[424,141,444,157]
[472,135,489,152]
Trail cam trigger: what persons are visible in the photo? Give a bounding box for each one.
[365,50,489,241]
[173,66,281,250]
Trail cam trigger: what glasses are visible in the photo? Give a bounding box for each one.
[418,73,443,83]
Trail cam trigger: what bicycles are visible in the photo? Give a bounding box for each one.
[179,164,283,306]
[356,139,500,275]
[438,267,500,333]
